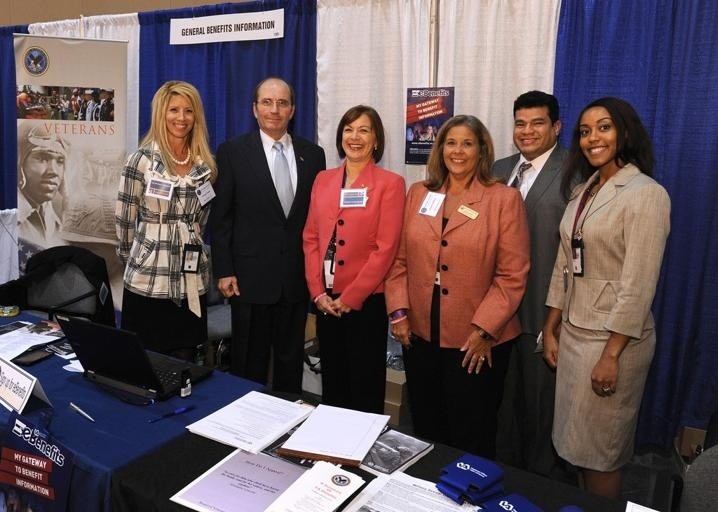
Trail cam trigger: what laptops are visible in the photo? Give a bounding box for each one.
[56,309,213,403]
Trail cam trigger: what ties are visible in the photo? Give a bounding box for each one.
[271,141,295,218]
[510,162,533,189]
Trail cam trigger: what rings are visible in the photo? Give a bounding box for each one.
[602,386,610,392]
[478,356,484,361]
[323,311,327,316]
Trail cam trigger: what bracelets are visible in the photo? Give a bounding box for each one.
[390,315,407,325]
[314,292,327,303]
[478,328,490,339]
[391,309,407,319]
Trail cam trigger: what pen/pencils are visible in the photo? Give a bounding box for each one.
[148,406,195,423]
[70,402,95,423]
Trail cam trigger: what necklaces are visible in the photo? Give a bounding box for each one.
[167,147,191,165]
[588,191,595,197]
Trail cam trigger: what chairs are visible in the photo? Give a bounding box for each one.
[680,444,718,510]
[0,246,115,326]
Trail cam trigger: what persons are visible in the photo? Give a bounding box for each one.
[541,96,673,501]
[412,127,425,142]
[115,79,219,345]
[208,76,326,395]
[382,114,532,456]
[406,124,414,142]
[419,124,436,142]
[301,104,407,413]
[17,84,117,249]
[488,89,588,474]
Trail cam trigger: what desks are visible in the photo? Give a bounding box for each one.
[1,271,266,512]
[110,389,627,510]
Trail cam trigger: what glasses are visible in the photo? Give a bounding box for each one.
[255,100,293,109]
[24,125,69,145]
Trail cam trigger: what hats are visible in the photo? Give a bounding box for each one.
[17,120,70,190]
